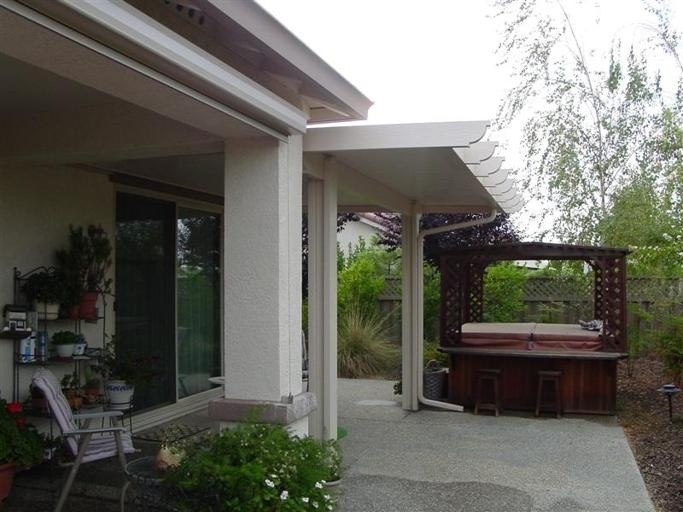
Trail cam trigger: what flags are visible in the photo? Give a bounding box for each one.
[0,463,17,503]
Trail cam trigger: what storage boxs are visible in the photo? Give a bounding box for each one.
[535,370,563,419]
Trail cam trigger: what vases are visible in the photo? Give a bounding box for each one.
[0,399,47,467]
[317,437,343,474]
[314,475,342,503]
[176,400,346,512]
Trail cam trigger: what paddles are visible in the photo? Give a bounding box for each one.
[132,422,210,443]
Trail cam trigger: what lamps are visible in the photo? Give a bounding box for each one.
[32,368,141,511]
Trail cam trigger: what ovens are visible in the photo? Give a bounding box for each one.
[460,321,602,352]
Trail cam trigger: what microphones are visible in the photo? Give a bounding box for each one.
[471,368,501,416]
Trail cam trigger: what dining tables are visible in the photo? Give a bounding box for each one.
[19,273,83,320]
[75,333,87,356]
[57,373,74,398]
[67,374,83,409]
[90,331,153,410]
[82,377,100,397]
[61,223,120,319]
[52,331,75,359]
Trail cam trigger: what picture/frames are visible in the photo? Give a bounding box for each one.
[438,348,620,415]
